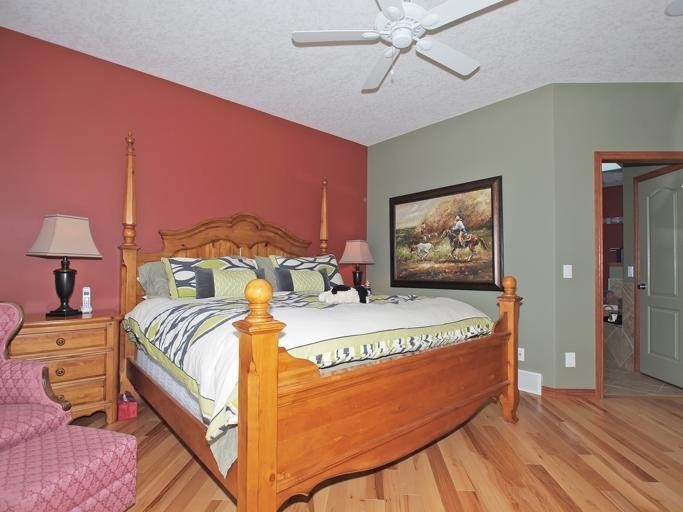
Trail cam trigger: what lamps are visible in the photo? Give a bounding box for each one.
[339,239,377,289]
[25,214,102,317]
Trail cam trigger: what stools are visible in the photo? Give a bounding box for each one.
[0,424,137,512]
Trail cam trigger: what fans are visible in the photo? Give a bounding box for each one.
[290,0,505,92]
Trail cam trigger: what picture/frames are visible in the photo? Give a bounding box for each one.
[388,174,504,292]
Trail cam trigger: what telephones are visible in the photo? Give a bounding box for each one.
[80,287,93,314]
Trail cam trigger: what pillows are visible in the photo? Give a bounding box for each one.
[137,253,344,300]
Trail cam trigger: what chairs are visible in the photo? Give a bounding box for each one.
[0,301,71,452]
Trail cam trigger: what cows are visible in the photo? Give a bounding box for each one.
[410,243,438,260]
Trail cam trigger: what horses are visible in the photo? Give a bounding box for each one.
[440,229,488,262]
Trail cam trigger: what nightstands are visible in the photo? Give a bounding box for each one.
[8,310,125,426]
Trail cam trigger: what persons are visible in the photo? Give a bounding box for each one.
[450,215,467,247]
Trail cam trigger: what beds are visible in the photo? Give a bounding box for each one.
[116,213,522,512]
